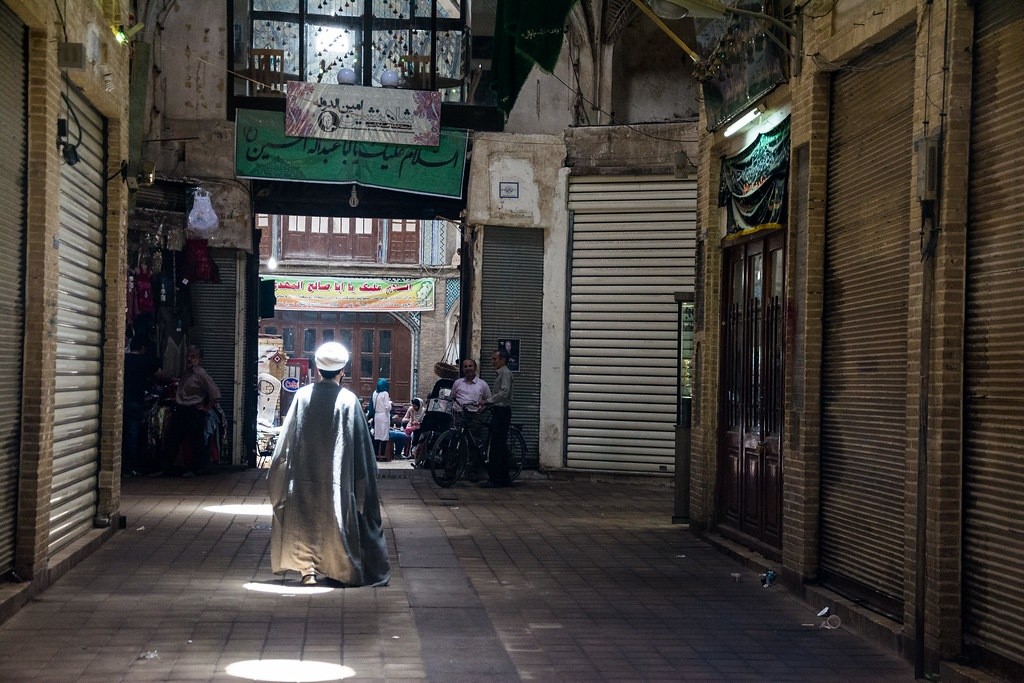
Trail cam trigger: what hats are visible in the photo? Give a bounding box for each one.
[315,341,350,372]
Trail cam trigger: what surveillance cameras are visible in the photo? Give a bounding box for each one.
[127,176,138,192]
[63,144,81,166]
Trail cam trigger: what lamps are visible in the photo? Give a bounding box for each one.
[338,68,355,83]
[381,69,401,88]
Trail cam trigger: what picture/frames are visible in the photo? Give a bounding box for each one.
[498,338,521,372]
[498,182,519,199]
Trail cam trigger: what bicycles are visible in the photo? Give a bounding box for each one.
[430,396,529,486]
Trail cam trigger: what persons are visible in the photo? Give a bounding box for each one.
[126,336,179,471]
[266,341,377,585]
[502,341,517,369]
[481,350,516,487]
[370,379,391,461]
[441,359,494,489]
[403,398,426,459]
[177,345,221,478]
[388,429,407,459]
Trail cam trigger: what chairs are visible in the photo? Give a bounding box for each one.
[249,47,287,95]
[401,56,439,92]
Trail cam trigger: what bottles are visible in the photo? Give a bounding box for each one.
[400,424,404,430]
[393,424,397,431]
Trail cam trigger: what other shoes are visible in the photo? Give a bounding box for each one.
[301,576,316,586]
[182,471,195,478]
[478,481,502,488]
[401,454,411,459]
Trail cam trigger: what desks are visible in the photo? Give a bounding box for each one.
[237,69,300,91]
[402,70,460,93]
